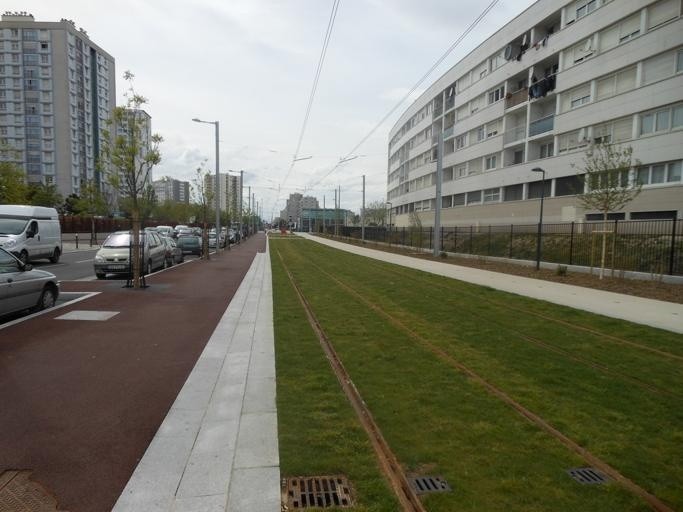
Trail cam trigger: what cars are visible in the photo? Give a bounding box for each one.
[0,247,59,323]
[88,224,244,279]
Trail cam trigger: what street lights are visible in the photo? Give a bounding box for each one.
[530,165,546,270]
[386,201,393,248]
[191,117,222,257]
[227,168,243,244]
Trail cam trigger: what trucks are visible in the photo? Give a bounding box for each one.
[0,203,63,265]
